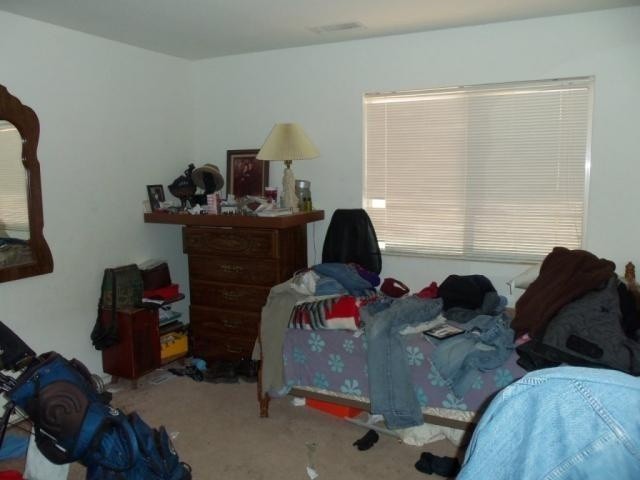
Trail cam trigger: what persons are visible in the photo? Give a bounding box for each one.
[243,161,256,179]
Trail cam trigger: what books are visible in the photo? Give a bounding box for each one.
[257,206,292,217]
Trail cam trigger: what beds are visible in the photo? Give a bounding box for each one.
[256,265,531,430]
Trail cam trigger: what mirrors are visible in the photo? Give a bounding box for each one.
[0,84,54,283]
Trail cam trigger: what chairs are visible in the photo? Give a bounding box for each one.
[322,208,382,274]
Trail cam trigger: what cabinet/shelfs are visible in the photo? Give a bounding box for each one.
[98,292,192,389]
[142,211,326,370]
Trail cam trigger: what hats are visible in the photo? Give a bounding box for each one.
[190,164,224,191]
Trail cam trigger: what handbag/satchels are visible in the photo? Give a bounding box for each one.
[102,263,144,309]
[89,317,121,349]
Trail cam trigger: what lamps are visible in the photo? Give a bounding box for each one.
[256,123,321,211]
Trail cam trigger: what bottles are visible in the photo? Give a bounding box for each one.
[295,179,312,211]
[263,186,277,204]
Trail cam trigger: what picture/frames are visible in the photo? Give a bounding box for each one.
[146,185,164,212]
[225,149,269,203]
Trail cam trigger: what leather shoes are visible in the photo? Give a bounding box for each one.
[237,357,257,382]
[204,359,238,383]
